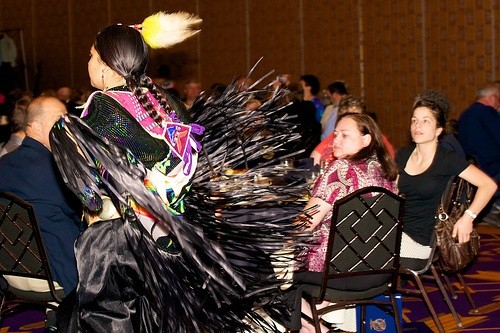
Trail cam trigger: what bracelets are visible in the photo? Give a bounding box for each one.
[465,209,477,219]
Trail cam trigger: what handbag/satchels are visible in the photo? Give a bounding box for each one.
[433,175,480,270]
[356,294,402,333]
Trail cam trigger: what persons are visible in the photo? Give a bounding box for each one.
[58,87,75,114]
[270,113,399,333]
[394,98,498,260]
[0,96,33,157]
[182,74,349,172]
[456,81,500,206]
[50,25,204,333]
[312,96,396,167]
[0,96,86,296]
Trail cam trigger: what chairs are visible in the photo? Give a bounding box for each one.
[0,192,71,333]
[291,161,481,333]
